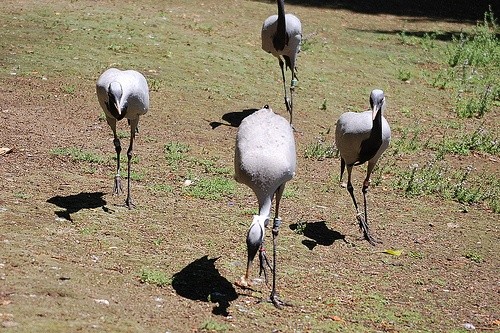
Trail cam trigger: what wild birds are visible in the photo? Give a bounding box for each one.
[233,108,296,310]
[96,68,149,210]
[261,0,302,132]
[334,90,391,247]
